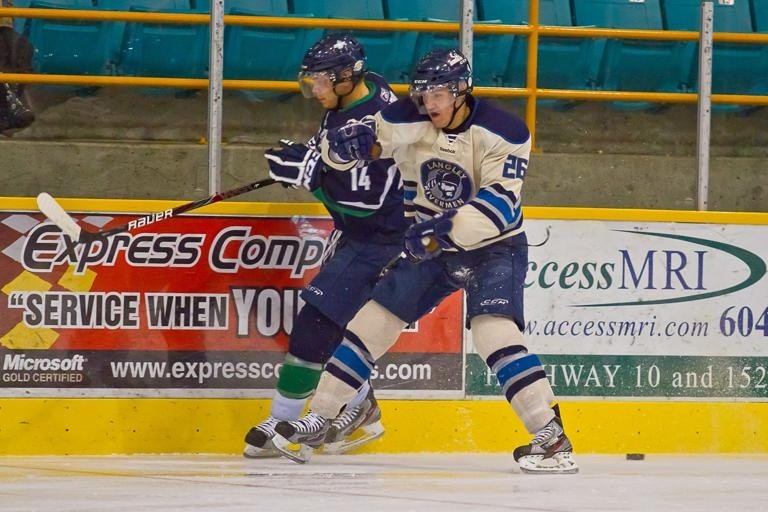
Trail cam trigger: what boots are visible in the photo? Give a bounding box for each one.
[513,404,573,462]
[325,382,382,443]
[275,402,346,450]
[245,417,282,450]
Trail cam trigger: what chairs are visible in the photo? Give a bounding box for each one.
[0,1,768,112]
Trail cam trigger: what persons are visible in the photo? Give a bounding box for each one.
[271,45,575,460]
[243,32,401,451]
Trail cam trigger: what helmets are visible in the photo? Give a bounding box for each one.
[298,35,367,99]
[408,48,473,115]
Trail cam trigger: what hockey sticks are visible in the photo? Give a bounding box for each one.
[36,179,279,242]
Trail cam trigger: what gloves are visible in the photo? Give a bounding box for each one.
[264,138,321,192]
[320,115,382,172]
[400,209,457,265]
